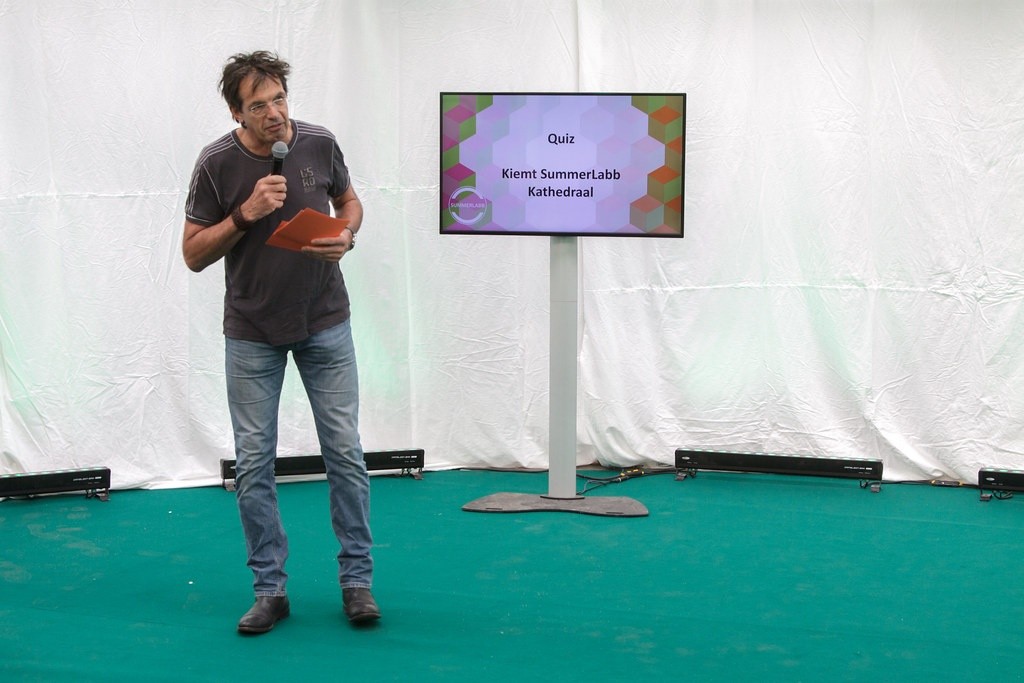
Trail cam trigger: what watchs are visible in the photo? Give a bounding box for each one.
[344,226,356,251]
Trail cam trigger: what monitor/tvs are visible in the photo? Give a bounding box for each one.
[439,92,687,239]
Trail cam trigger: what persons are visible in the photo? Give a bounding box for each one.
[182,50,381,634]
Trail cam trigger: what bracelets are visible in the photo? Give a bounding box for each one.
[231,205,254,231]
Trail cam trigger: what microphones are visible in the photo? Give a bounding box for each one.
[271,141,289,177]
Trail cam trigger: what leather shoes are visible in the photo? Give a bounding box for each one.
[238,596,290,631]
[343,588,381,622]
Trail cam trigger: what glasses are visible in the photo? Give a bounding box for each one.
[239,98,287,120]
